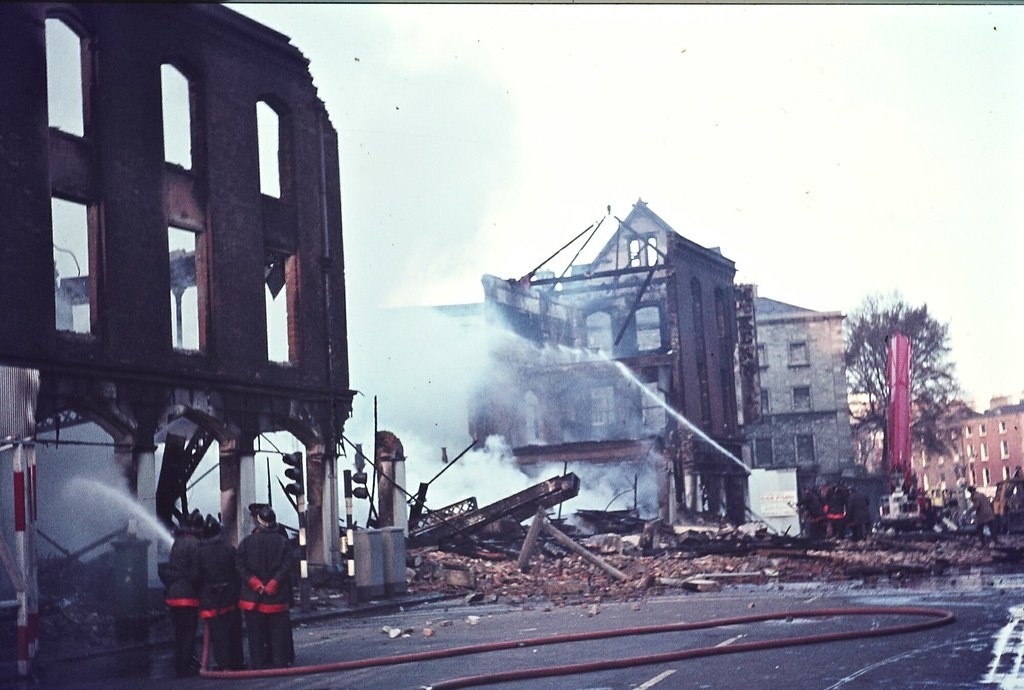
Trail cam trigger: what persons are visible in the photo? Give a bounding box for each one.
[965,486,999,546]
[796,475,872,540]
[161,504,297,679]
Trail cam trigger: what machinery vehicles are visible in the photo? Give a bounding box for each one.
[992,465,1024,535]
[871,484,930,536]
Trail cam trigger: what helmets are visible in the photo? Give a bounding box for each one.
[175,509,204,537]
[256,506,279,532]
[201,514,224,544]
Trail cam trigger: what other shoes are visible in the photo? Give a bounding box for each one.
[178,655,202,678]
